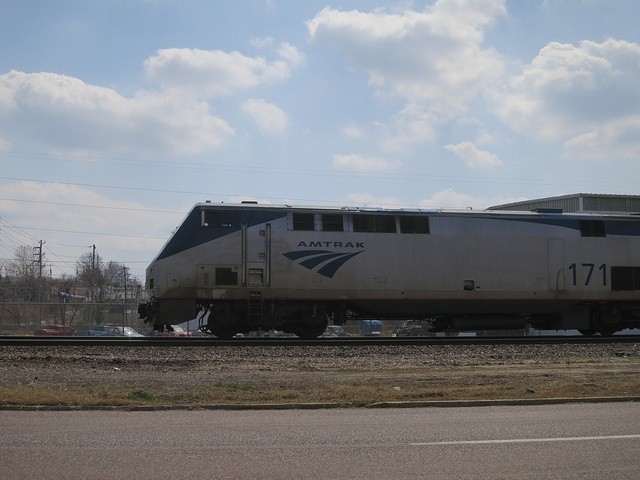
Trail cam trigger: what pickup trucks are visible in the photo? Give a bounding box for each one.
[35,325,74,336]
[88,326,110,335]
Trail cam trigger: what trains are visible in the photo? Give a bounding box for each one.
[138,200,640,339]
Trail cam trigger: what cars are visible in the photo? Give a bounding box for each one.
[109,327,144,336]
[155,325,192,336]
[328,326,349,336]
[396,320,436,337]
